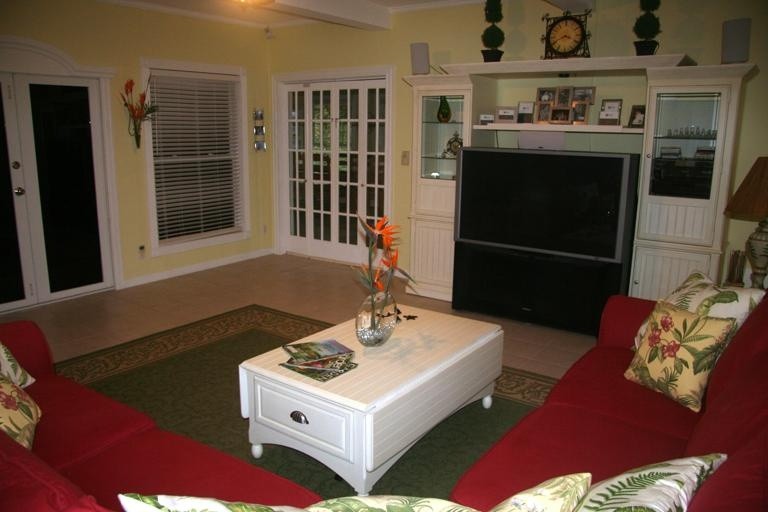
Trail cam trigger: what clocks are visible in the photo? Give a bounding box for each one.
[538,9,592,58]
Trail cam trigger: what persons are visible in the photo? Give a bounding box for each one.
[541,91,554,101]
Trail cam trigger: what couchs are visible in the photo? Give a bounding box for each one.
[1,293,768,511]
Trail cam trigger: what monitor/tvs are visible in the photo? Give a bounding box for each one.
[453,145,632,264]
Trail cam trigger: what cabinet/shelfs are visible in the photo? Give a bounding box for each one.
[401,73,497,303]
[626,61,761,302]
[453,66,640,341]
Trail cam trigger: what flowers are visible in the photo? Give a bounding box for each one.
[117,74,161,148]
[348,208,418,331]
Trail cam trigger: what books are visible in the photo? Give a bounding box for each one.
[661,147,681,159]
[278,340,358,383]
[695,146,715,160]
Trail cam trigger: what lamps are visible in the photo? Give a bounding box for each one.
[723,156,768,289]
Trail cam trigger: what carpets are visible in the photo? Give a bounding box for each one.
[47,300,562,500]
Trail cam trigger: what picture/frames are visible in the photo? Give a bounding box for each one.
[479,82,644,129]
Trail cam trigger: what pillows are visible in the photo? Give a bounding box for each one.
[625,297,736,413]
[0,373,42,452]
[116,493,306,510]
[300,496,478,512]
[634,270,766,353]
[488,473,591,512]
[575,453,726,512]
[1,341,36,389]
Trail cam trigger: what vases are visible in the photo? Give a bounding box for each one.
[354,291,399,346]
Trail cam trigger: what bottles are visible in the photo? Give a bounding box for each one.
[447,131,463,156]
[437,96,452,123]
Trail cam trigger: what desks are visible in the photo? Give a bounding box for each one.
[236,304,506,497]
